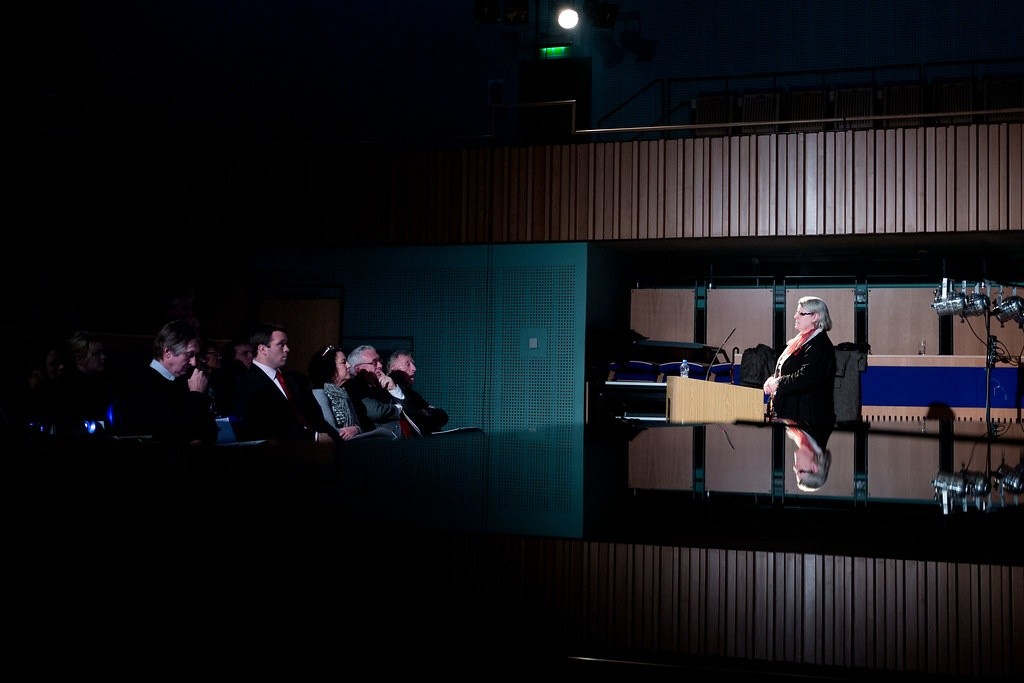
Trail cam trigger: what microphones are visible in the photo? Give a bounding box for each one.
[716,424,734,450]
[705,327,737,381]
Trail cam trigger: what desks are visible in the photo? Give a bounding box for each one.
[860,353,1024,423]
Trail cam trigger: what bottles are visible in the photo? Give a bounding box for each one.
[680,359,689,378]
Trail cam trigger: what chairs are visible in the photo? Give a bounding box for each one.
[832,344,867,421]
[609,360,771,404]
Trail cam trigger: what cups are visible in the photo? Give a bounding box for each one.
[918,421,926,433]
[917,340,926,355]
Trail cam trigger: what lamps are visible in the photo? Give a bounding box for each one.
[992,286,1024,326]
[931,278,965,317]
[958,282,991,319]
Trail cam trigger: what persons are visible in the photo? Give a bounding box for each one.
[777,418,836,491]
[14,292,447,444]
[764,298,836,418]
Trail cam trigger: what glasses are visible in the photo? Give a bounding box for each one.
[357,359,383,365]
[322,344,336,356]
[795,310,814,318]
[203,351,220,357]
[795,469,813,475]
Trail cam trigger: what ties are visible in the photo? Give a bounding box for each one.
[275,370,313,431]
